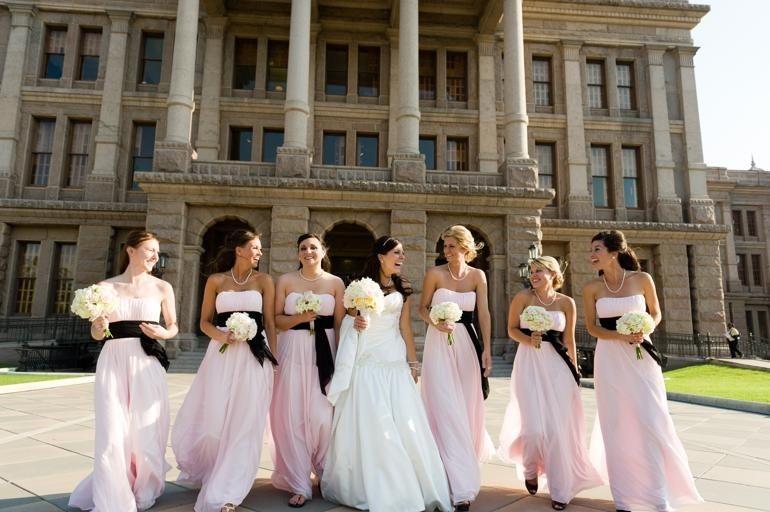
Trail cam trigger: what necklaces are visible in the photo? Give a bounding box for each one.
[298,267,325,281]
[382,275,392,287]
[602,268,626,293]
[447,261,469,281]
[535,289,557,306]
[230,267,254,286]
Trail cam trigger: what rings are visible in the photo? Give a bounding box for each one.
[487,367,491,370]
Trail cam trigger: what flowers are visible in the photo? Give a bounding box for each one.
[70,283,119,339]
[616,311,656,361]
[217,312,260,354]
[295,290,323,336]
[520,305,553,350]
[343,276,386,334]
[429,301,463,345]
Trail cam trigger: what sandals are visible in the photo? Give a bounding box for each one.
[221,504,237,511]
[288,494,305,507]
[552,502,566,511]
[526,480,538,495]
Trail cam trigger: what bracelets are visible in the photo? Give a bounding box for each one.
[408,360,419,371]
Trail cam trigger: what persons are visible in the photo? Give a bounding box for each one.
[726,322,745,359]
[270,233,346,508]
[320,236,455,511]
[68,230,180,512]
[582,228,705,512]
[416,224,493,512]
[172,228,278,512]
[499,255,605,510]
